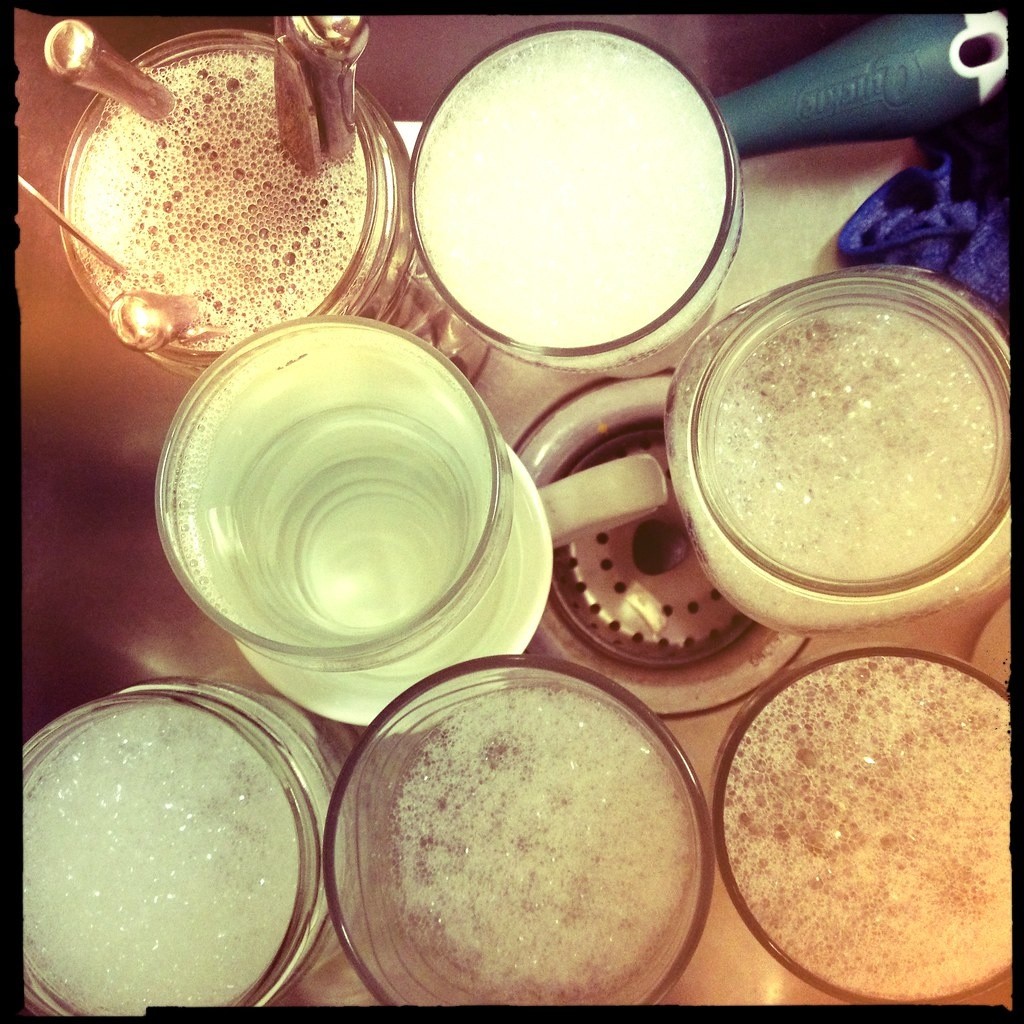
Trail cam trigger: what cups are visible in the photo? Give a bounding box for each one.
[59,29,411,372]
[714,646,1013,1001]
[154,317,515,670]
[662,268,1012,637]
[408,20,742,372]
[322,652,713,1007]
[22,682,345,1021]
[234,440,669,724]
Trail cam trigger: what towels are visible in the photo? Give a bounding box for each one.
[835,97,1011,325]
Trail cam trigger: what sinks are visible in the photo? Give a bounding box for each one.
[11,4,1015,1012]
